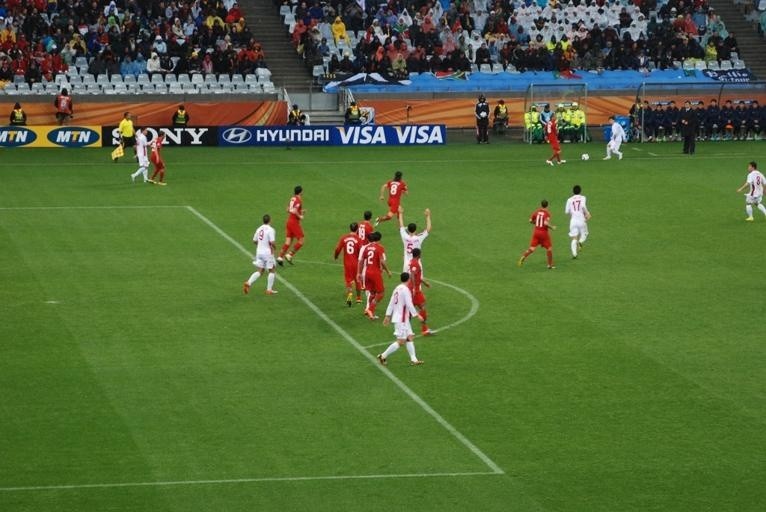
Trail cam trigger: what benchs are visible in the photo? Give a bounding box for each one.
[92,73,277,94]
[347,30,365,48]
[0,56,92,95]
[280,5,296,33]
[312,64,324,76]
[649,10,659,17]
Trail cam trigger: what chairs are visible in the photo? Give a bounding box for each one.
[469,63,519,73]
[672,60,745,69]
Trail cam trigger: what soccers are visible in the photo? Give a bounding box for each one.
[582,153,589,161]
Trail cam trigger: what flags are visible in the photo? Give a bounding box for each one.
[110,145,124,161]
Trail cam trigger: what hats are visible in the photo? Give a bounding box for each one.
[497,99,504,105]
[530,102,579,110]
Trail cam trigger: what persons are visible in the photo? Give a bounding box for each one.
[375,171,409,226]
[112,112,141,164]
[377,272,426,366]
[736,162,766,223]
[543,113,568,167]
[128,126,149,186]
[242,215,281,295]
[563,184,592,260]
[396,204,433,290]
[517,199,559,270]
[148,131,168,185]
[276,186,307,268]
[355,231,393,321]
[357,233,387,311]
[355,210,375,246]
[402,247,438,336]
[334,222,363,308]
[679,100,698,155]
[603,116,628,162]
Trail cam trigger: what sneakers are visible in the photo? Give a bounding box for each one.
[243,254,296,295]
[374,216,381,228]
[745,215,753,221]
[532,134,762,167]
[346,298,437,366]
[114,157,166,186]
[518,241,583,269]
